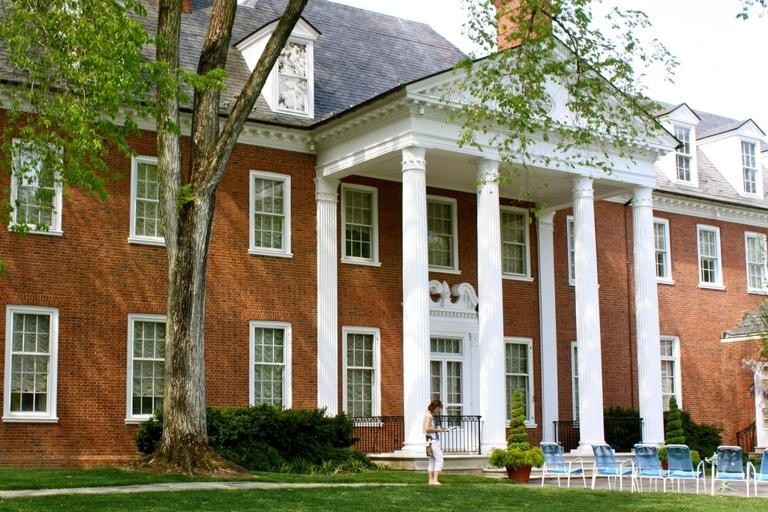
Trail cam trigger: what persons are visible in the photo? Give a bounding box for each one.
[423,399,450,486]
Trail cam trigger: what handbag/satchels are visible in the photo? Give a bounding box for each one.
[426,443,433,457]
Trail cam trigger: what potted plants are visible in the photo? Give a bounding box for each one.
[486,445,545,484]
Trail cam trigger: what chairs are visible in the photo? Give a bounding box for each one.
[539,440,768,499]
